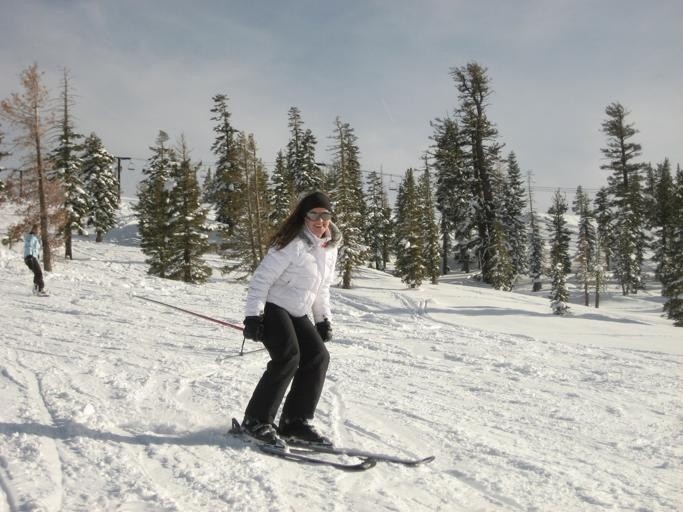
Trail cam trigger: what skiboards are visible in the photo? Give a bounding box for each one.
[228,418,435,471]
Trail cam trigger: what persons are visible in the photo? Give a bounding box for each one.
[24,223,48,294]
[241,192,344,455]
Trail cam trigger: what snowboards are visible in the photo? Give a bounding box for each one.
[32,288,48,297]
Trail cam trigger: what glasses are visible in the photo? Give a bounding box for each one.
[305,210,332,221]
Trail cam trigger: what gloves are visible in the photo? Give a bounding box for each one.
[317,322,332,342]
[243,316,264,342]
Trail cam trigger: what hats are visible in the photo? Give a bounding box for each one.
[303,191,330,211]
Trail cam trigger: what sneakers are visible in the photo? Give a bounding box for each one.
[240,421,289,451]
[278,410,331,446]
